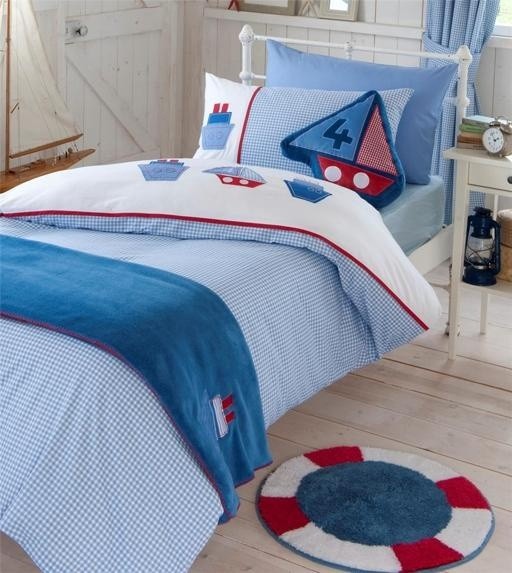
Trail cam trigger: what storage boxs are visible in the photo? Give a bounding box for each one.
[497,209,512,283]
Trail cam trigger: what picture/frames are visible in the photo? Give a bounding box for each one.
[318,0,358,20]
[237,0,298,16]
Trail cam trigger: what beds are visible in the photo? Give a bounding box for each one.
[0,23,474,573]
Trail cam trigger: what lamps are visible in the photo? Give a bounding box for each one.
[463,206,502,286]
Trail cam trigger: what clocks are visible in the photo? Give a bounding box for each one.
[480,116,512,160]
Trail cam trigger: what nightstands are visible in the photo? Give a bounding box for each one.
[443,146,512,364]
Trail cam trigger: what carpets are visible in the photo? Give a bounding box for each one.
[256,445,494,572]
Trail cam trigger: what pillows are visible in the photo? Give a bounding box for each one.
[192,69,415,200]
[265,38,456,183]
